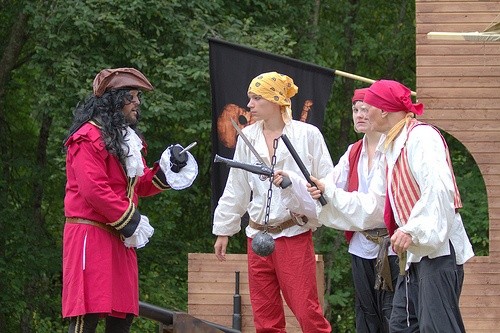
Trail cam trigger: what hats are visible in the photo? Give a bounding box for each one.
[93,67,154,97]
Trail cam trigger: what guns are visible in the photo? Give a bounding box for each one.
[214,154,292,189]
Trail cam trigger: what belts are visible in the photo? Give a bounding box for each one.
[366,228,390,237]
[66,217,118,234]
[248,214,309,235]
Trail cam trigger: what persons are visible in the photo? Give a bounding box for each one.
[270,88,399,333]
[212,71,338,333]
[307,80,475,333]
[62,68,199,333]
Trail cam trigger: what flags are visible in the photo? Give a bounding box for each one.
[206,37,337,209]
